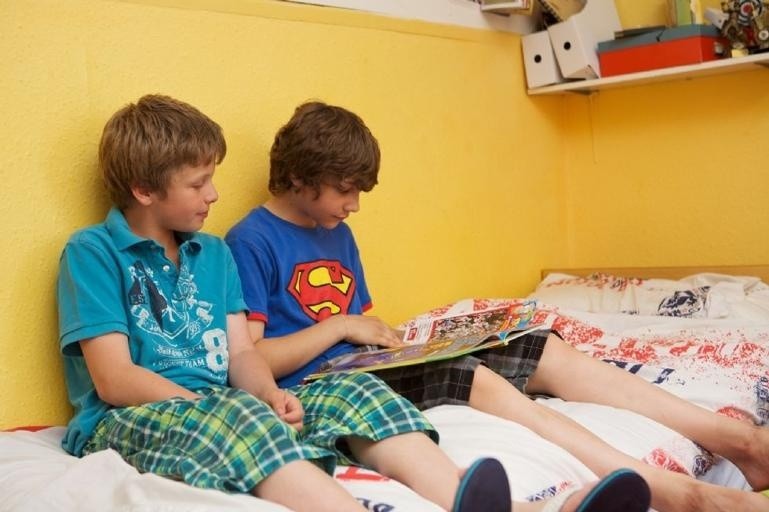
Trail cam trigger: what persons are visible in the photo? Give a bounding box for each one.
[221,100,769,512]
[55,93,653,511]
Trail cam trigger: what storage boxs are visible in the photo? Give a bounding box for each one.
[520,15,728,90]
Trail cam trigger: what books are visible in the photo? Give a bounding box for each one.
[303,298,549,381]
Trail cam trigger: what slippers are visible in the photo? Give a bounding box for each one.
[542,467,652,512]
[451,456,512,512]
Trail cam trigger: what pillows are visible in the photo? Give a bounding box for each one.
[528,272,769,318]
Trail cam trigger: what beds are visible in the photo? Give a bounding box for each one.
[0,267,769,512]
